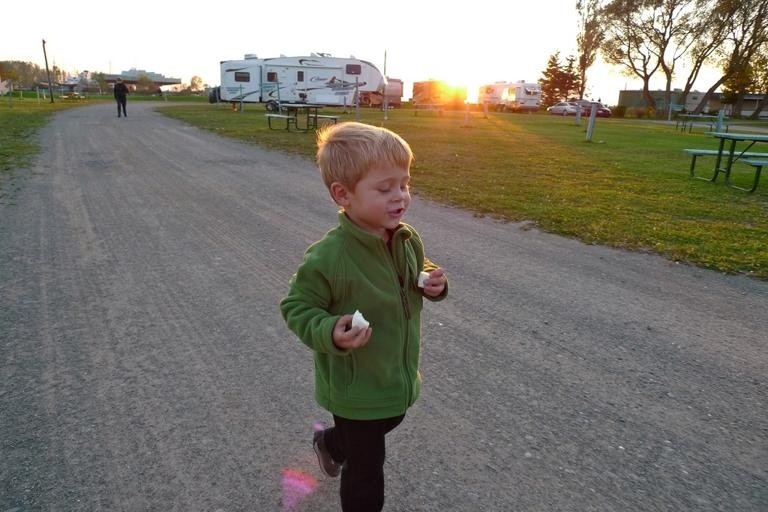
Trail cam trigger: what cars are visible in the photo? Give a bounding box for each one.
[547,101,611,117]
[209,86,220,104]
[60,78,101,94]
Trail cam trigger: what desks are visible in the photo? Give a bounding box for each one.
[679,115,729,132]
[280,103,323,133]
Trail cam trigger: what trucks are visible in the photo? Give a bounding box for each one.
[358,79,542,114]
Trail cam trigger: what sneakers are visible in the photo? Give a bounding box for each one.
[311,429,341,479]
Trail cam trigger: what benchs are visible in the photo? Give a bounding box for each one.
[675,118,768,193]
[264,114,341,133]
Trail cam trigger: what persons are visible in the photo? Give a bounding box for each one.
[276,120,451,511]
[113,77,129,118]
